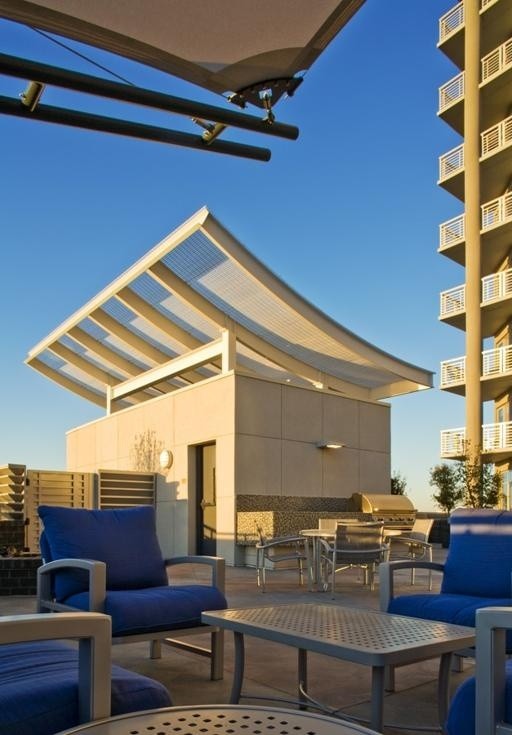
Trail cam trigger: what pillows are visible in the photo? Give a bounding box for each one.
[37,504,169,602]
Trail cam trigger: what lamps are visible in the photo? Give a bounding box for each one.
[159,451,172,471]
[316,440,348,449]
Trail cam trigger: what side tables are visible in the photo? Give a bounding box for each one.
[57,703,382,734]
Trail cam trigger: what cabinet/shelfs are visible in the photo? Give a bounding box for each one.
[24,471,100,551]
[97,469,156,509]
[0,463,26,521]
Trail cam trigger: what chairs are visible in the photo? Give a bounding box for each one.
[0,611,172,735]
[36,506,226,680]
[377,509,512,693]
[441,603,512,735]
[254,517,435,592]
